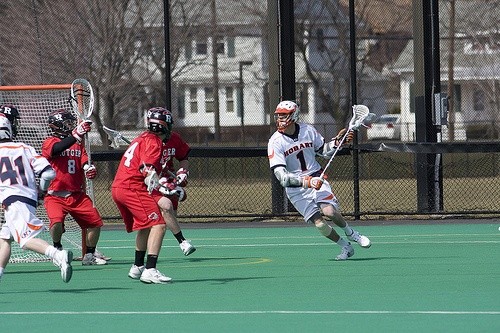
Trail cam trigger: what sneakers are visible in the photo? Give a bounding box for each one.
[82,252,106,265]
[179,240,196,256]
[345,229,372,248]
[53,250,73,282]
[335,242,354,260]
[128,264,146,279]
[140,268,172,284]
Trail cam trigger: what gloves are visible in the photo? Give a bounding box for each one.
[175,168,190,186]
[71,120,92,143]
[302,176,324,191]
[331,129,354,146]
[156,176,177,195]
[84,164,97,179]
[174,186,187,202]
[37,187,47,206]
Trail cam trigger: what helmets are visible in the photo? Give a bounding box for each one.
[274,101,299,132]
[0,116,13,143]
[48,109,77,141]
[0,104,19,137]
[147,107,174,138]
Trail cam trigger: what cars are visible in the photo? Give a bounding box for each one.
[366,114,401,140]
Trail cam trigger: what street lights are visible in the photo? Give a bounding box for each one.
[239,61,254,145]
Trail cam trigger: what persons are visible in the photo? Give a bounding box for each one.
[0,104,73,283]
[111,106,186,285]
[266,101,373,260]
[42,109,107,267]
[152,132,196,256]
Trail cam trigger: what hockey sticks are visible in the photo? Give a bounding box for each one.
[69,76,99,210]
[317,104,376,184]
[101,125,179,180]
